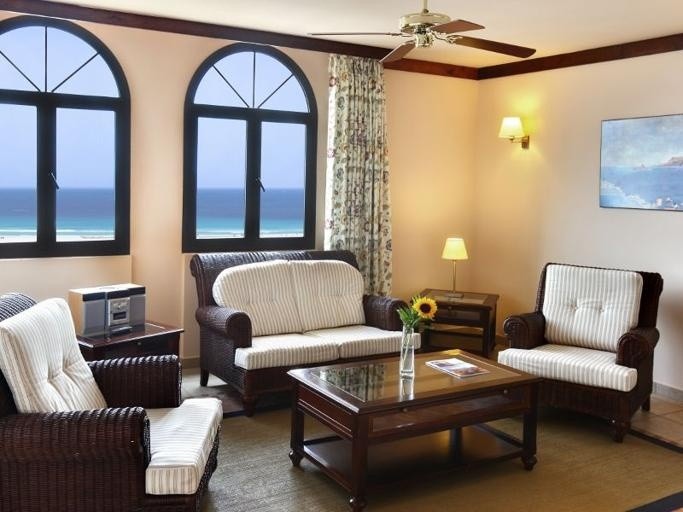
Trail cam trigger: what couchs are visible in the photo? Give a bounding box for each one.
[189,249,410,417]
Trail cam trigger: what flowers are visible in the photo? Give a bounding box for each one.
[394,296,438,360]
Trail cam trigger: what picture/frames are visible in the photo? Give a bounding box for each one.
[599,114,683,212]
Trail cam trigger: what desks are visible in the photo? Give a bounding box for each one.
[410,287,499,357]
[77,317,185,362]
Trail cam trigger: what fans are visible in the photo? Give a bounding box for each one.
[301,0,534,68]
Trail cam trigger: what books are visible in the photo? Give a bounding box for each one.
[425,357,490,379]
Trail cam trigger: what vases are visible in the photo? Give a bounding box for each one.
[399,327,414,376]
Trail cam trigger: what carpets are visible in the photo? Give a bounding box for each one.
[197,408,682,512]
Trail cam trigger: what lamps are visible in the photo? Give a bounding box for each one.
[497,115,528,149]
[440,237,468,298]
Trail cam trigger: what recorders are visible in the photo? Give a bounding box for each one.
[67,283,147,338]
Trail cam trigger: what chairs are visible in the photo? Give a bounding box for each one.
[495,262,662,444]
[1,289,226,512]
